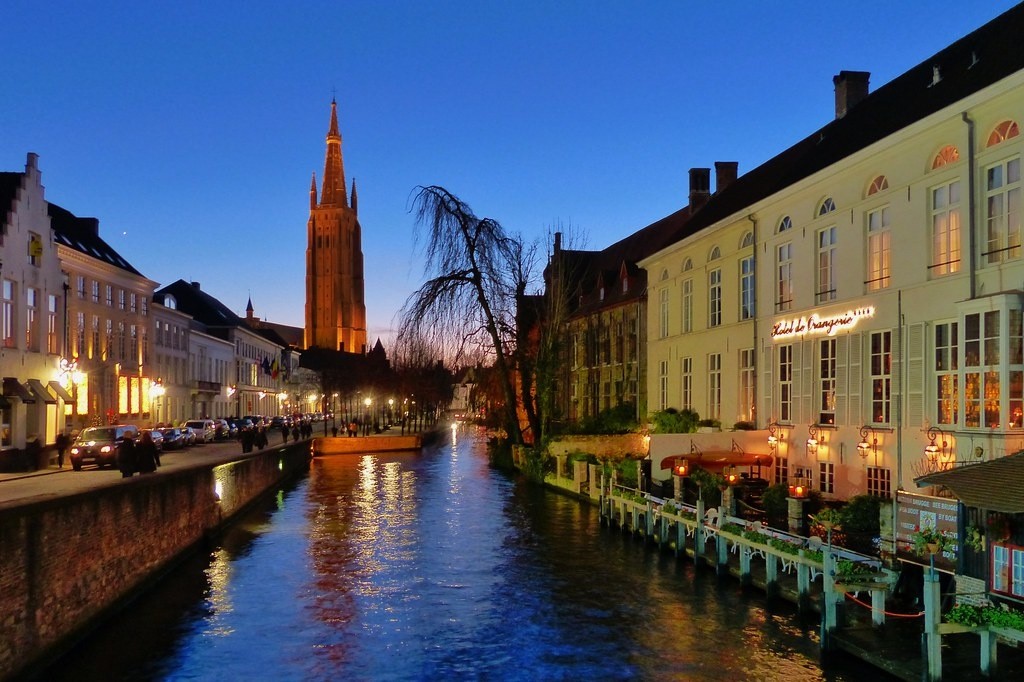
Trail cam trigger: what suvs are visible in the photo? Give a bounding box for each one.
[69,424,141,472]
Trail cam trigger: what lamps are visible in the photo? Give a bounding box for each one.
[857,425,878,459]
[924,426,948,463]
[766,423,784,450]
[788,483,807,498]
[806,423,824,454]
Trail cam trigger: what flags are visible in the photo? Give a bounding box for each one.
[264,357,288,379]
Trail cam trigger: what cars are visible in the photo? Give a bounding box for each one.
[136,409,334,452]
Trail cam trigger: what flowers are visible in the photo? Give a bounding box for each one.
[904,522,949,558]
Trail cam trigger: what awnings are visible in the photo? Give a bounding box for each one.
[661,438,773,470]
[49,381,75,404]
[28,379,56,404]
[3,377,35,403]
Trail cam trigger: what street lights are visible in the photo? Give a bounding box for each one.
[388,398,394,427]
[365,397,372,435]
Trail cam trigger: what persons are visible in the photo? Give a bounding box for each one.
[56,430,67,467]
[237,425,268,453]
[119,431,161,478]
[157,420,178,427]
[282,422,313,442]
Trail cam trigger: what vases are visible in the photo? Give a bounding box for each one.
[925,542,939,554]
